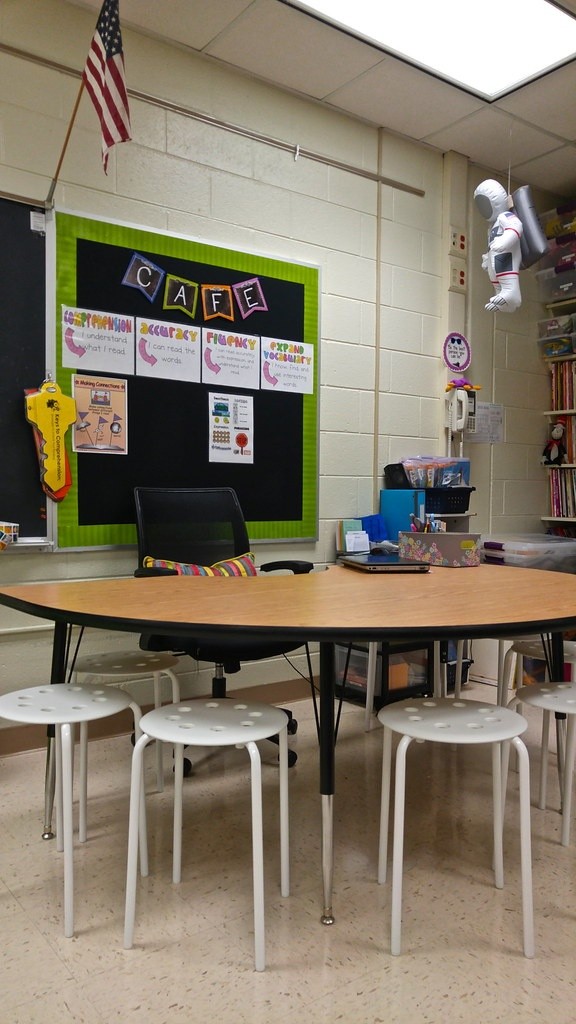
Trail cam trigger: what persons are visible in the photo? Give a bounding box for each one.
[472,179,525,313]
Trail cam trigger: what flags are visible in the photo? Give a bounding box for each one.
[84,0,134,176]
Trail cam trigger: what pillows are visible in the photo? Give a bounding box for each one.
[143,551,257,576]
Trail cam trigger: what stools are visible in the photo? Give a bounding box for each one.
[491,681,576,870]
[69,649,180,842]
[376,697,536,960]
[121,699,291,972]
[455,632,552,707]
[0,682,148,940]
[499,639,575,772]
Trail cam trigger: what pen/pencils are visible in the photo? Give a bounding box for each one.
[409,512,447,532]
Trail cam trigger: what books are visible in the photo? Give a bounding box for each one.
[547,362,576,538]
[335,520,370,555]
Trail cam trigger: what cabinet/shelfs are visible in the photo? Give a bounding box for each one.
[335,641,435,714]
[535,294,576,524]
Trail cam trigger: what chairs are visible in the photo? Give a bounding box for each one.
[126,487,315,777]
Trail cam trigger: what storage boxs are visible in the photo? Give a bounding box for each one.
[336,644,429,695]
[447,658,475,687]
[537,313,576,358]
[479,534,576,574]
[409,485,477,515]
[535,204,576,303]
[397,530,481,568]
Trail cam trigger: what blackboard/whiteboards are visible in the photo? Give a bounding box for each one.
[0,190,58,546]
[57,210,322,548]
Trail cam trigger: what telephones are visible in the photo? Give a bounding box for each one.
[444,387,477,435]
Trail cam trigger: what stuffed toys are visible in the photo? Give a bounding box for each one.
[542,420,569,466]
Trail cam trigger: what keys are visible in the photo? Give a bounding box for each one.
[25,383,77,492]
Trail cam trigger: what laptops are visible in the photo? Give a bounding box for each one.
[338,555,430,574]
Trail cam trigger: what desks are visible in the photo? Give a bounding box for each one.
[0,562,576,926]
